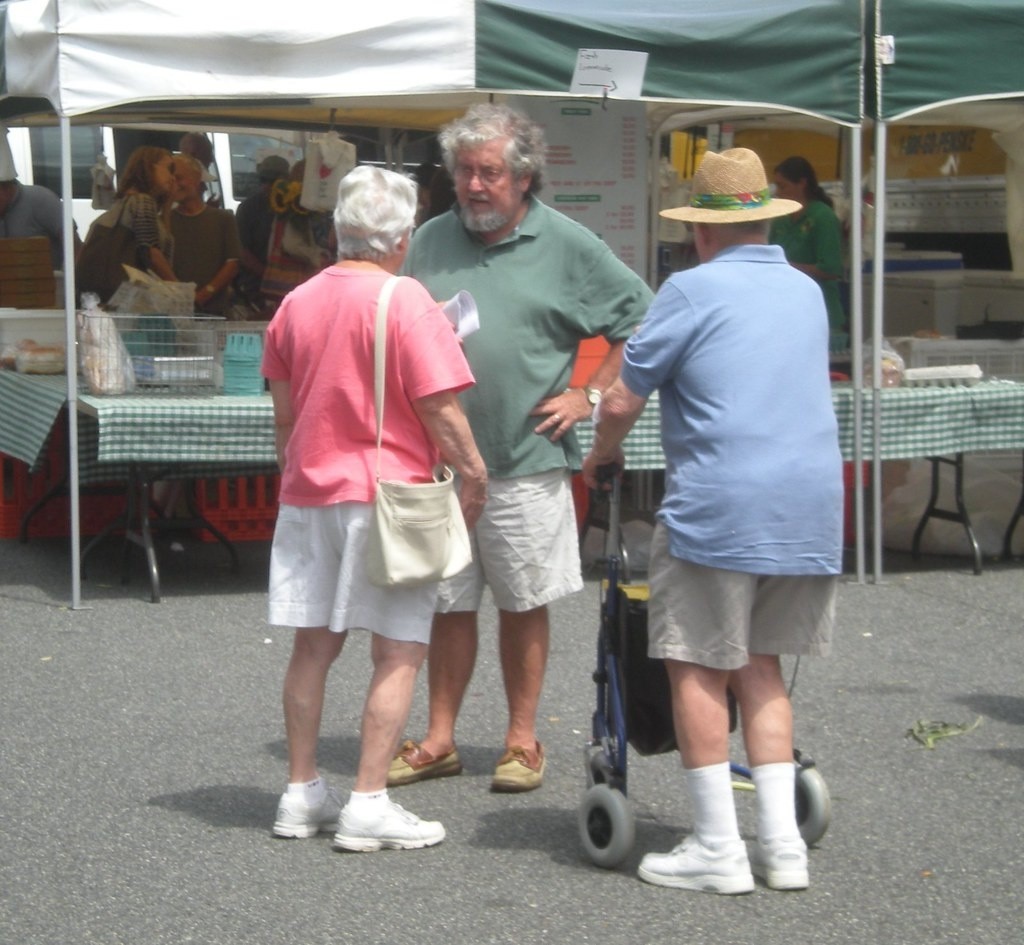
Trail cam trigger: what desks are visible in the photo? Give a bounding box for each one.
[0,364,1024,603]
[865,270,1023,377]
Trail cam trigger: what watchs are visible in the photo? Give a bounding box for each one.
[584,385,603,406]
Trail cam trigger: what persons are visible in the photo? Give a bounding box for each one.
[1,180,83,275]
[772,153,849,351]
[83,134,294,317]
[261,165,488,848]
[389,103,653,791]
[583,146,846,893]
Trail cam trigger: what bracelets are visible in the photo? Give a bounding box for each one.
[205,284,215,296]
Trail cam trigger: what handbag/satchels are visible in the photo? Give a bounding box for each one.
[368,464,473,586]
[259,217,309,295]
[77,195,134,298]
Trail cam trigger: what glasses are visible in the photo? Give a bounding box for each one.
[456,166,511,182]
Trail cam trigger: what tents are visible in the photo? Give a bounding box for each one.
[873,0,1023,578]
[3,0,869,607]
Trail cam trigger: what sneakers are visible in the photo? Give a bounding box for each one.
[274,792,344,839]
[745,833,809,889]
[333,804,445,853]
[638,834,755,894]
[388,741,462,784]
[491,739,546,790]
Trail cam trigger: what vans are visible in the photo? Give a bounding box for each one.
[0,121,302,242]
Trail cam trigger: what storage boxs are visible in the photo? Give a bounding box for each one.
[0,235,281,539]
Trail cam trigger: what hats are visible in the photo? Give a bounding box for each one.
[257,156,290,176]
[659,148,803,224]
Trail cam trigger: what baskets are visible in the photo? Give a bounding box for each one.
[74,311,228,397]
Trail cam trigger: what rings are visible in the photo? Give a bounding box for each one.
[554,414,561,422]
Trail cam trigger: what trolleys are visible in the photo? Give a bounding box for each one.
[575,453,836,871]
